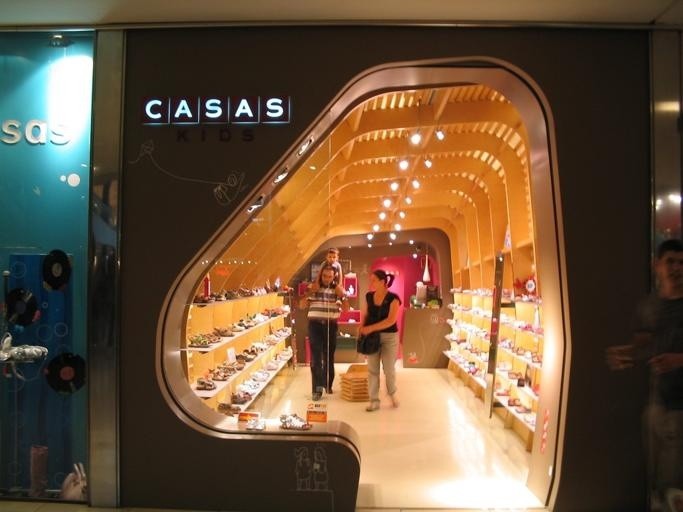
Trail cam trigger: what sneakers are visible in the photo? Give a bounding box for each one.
[312,388,332,401]
[336,299,342,305]
[445,287,544,426]
[307,295,317,301]
[366,400,380,411]
[390,391,400,408]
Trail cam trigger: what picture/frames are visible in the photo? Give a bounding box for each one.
[309,261,322,282]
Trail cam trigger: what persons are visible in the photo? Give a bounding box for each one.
[298,265,351,401]
[355,269,403,411]
[608,238,683,512]
[305,247,348,306]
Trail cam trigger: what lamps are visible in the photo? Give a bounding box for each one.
[423,240,430,282]
[366,99,444,248]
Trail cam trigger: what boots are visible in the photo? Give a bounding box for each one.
[29,444,53,498]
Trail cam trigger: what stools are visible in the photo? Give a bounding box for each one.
[339,364,370,402]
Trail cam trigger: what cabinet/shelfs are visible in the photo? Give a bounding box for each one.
[442,289,543,451]
[187,291,297,418]
[298,277,361,324]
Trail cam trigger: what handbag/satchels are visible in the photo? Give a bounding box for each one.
[60,462,87,501]
[356,331,379,354]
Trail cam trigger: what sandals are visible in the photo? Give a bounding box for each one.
[246,417,265,430]
[187,281,293,417]
[280,414,312,430]
